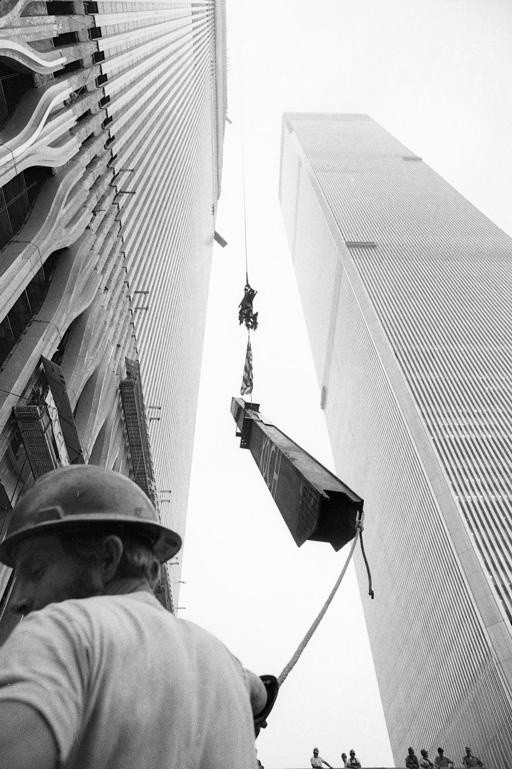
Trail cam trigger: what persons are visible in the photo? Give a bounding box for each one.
[310,747,334,768]
[405,746,456,769]
[0,463,268,769]
[462,746,486,769]
[341,749,361,769]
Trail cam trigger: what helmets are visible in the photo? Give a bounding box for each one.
[408,746,471,754]
[0,464,182,569]
[313,748,356,758]
